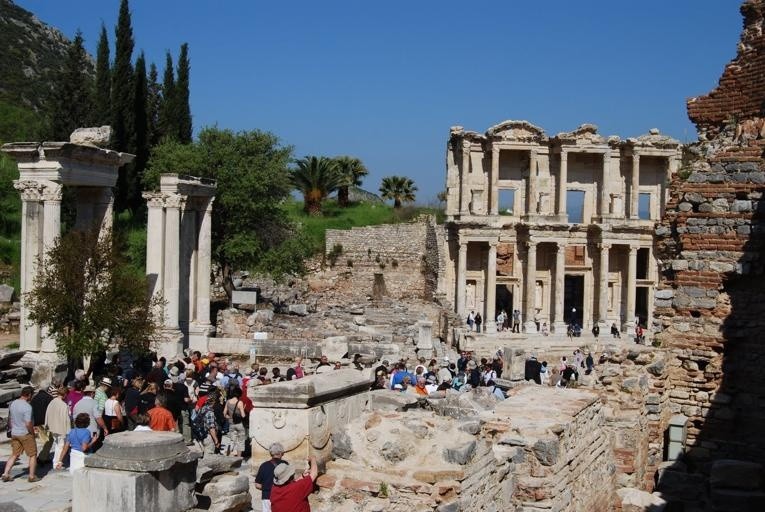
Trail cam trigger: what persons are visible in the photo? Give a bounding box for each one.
[371,350,506,400]
[316,354,364,372]
[255,443,288,512]
[468,309,642,344]
[3,348,304,482]
[524,349,609,389]
[270,456,318,512]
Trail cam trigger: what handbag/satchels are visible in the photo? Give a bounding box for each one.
[231,399,242,425]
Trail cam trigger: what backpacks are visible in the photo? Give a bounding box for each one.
[224,374,240,390]
[190,405,212,442]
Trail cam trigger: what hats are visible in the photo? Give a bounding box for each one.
[272,463,296,486]
[83,385,97,392]
[163,366,186,384]
[467,360,477,369]
[100,376,113,388]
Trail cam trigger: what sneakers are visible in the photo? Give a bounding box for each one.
[1,474,14,482]
[28,474,41,482]
